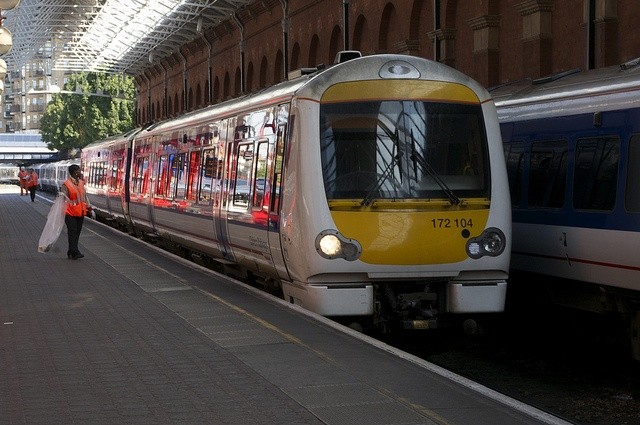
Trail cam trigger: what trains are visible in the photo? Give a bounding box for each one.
[29,50,513,317]
[497,56,640,291]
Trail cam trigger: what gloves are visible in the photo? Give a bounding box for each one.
[90,210,96,220]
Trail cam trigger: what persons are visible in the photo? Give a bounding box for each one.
[61,164,97,260]
[26,168,38,202]
[17,166,28,196]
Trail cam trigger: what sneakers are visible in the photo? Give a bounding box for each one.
[67,249,84,260]
